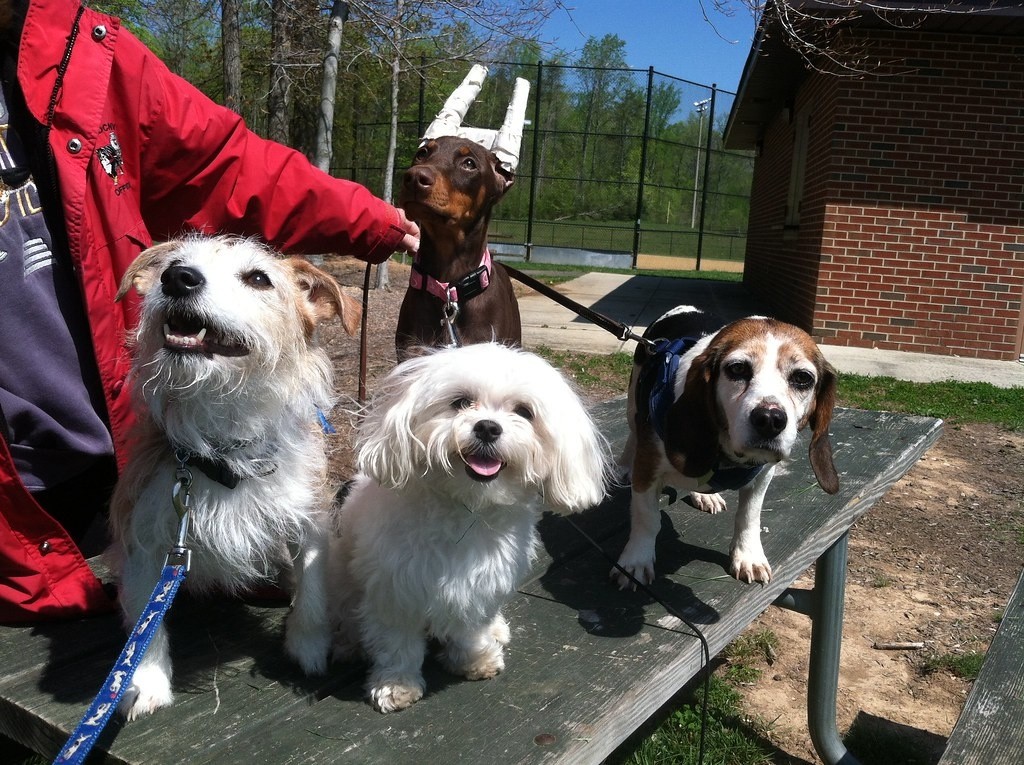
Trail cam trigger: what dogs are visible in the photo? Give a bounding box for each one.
[607,305,843,593]
[318,323,619,714]
[395,62,530,362]
[106,227,364,722]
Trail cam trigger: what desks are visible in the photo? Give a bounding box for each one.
[0,390,944,765]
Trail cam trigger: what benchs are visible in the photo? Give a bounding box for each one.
[938,568,1024,765]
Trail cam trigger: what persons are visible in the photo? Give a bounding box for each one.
[0,0,421,620]
